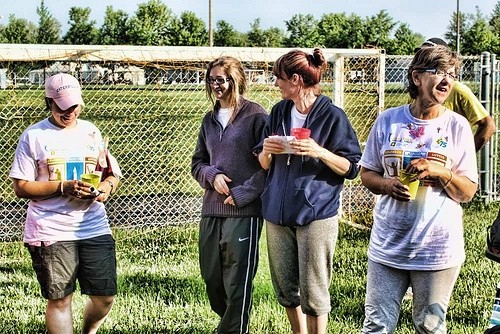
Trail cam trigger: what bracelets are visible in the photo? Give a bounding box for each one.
[105,180,113,193]
[60,181,64,195]
[442,170,454,189]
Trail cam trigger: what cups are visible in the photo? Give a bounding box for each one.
[399,169,420,200]
[80,173,99,190]
[291,128,311,139]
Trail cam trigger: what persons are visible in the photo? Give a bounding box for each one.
[406,36,496,155]
[190,56,270,334]
[354,43,479,334]
[8,73,126,334]
[250,48,362,334]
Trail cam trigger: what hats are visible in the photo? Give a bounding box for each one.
[45,72,85,110]
[414,37,449,54]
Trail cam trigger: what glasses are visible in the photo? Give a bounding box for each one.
[206,76,230,85]
[422,67,455,81]
